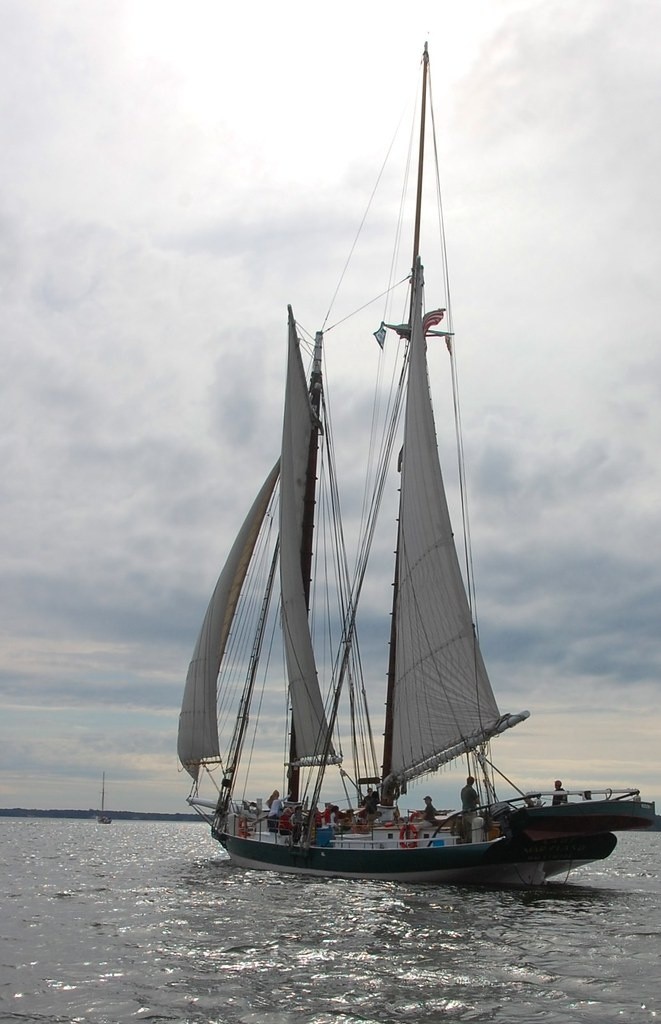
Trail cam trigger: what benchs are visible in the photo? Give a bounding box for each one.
[334,832,373,848]
[257,830,293,844]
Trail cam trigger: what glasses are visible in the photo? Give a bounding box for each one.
[368,791,372,792]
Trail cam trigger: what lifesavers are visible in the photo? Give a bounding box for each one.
[399,824,420,848]
[238,816,247,838]
[410,813,419,822]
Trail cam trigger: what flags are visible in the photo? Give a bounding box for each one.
[422,311,443,334]
[374,326,386,348]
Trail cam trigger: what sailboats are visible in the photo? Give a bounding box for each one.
[175,41,655,890]
[99,771,112,824]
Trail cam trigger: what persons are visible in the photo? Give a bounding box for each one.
[361,788,380,814]
[280,805,339,841]
[461,777,480,842]
[266,789,292,832]
[552,780,568,806]
[416,796,438,830]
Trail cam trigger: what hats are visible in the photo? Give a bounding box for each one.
[367,788,372,791]
[423,796,432,801]
